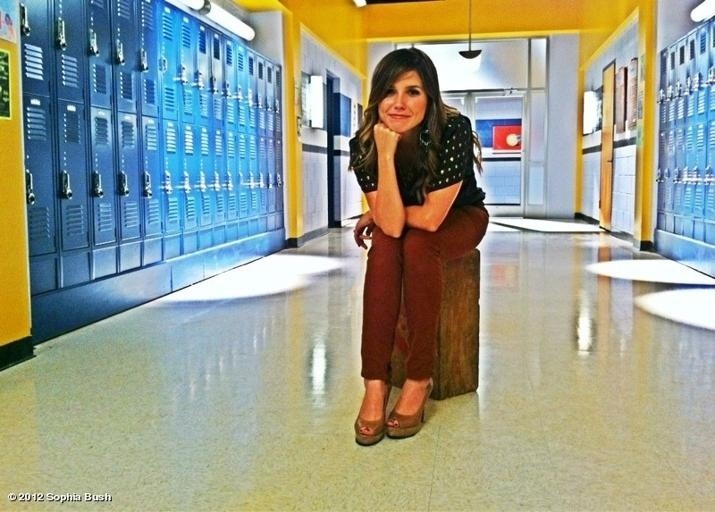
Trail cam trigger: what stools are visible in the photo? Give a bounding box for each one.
[377,242,488,406]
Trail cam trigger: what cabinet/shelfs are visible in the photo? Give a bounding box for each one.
[655,12,715,282]
[20,0,285,337]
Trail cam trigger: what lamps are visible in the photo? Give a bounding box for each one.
[307,74,328,130]
[458,0,484,73]
[581,89,594,136]
[689,1,715,25]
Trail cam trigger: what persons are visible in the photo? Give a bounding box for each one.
[345,49,488,446]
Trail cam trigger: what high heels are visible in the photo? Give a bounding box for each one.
[385,377,434,438]
[354,381,392,445]
